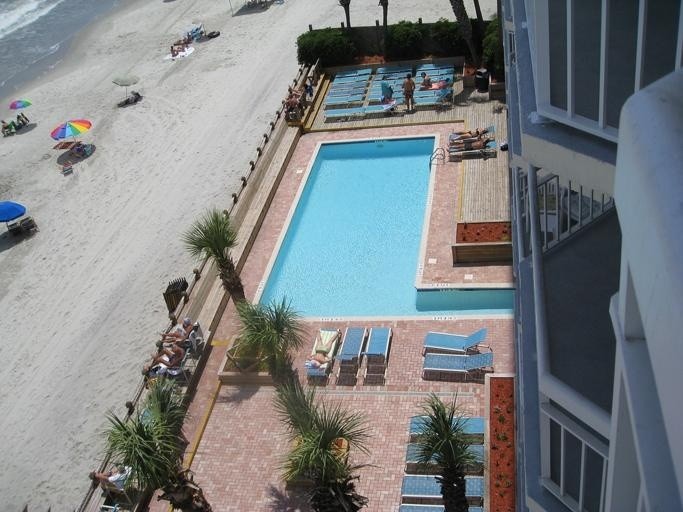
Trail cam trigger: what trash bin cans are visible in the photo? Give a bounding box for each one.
[163,277,188,312]
[474,68,489,91]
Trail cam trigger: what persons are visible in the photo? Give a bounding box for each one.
[89,462,127,488]
[156,317,193,346]
[130,90,140,102]
[402,74,415,111]
[0,120,8,137]
[380,75,394,100]
[150,345,185,369]
[286,85,301,98]
[451,127,489,140]
[169,32,192,57]
[303,83,313,100]
[448,137,490,150]
[283,95,299,115]
[419,71,439,88]
[305,327,341,370]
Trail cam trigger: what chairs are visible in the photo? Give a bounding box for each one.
[306,326,492,512]
[96,322,212,512]
[324,64,496,163]
[9,217,37,235]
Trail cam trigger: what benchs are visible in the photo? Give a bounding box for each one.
[283,58,321,124]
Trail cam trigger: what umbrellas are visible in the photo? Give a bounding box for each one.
[0,201,27,223]
[111,73,140,101]
[8,100,32,112]
[49,118,91,147]
